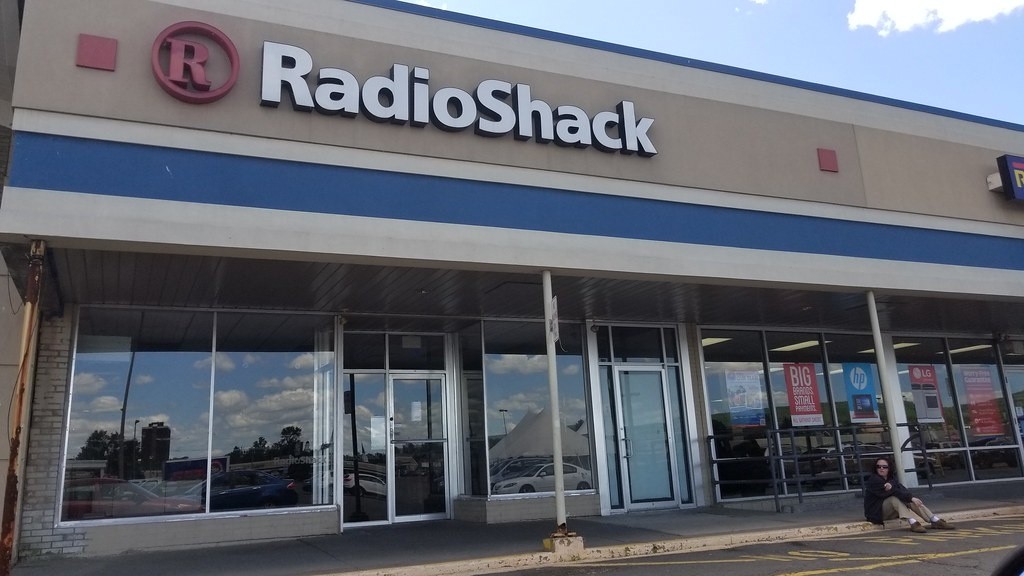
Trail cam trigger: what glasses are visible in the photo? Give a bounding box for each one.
[876,465,889,468]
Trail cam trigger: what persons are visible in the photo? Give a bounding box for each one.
[927,426,938,442]
[863,457,956,534]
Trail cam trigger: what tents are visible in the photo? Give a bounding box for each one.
[490,407,591,470]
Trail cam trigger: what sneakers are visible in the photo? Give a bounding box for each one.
[911,521,927,533]
[931,519,956,529]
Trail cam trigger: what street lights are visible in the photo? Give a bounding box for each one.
[499,410,508,435]
[132,420,140,479]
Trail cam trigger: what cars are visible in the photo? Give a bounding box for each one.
[433,458,592,498]
[757,435,1024,487]
[62,469,299,521]
[304,468,388,500]
